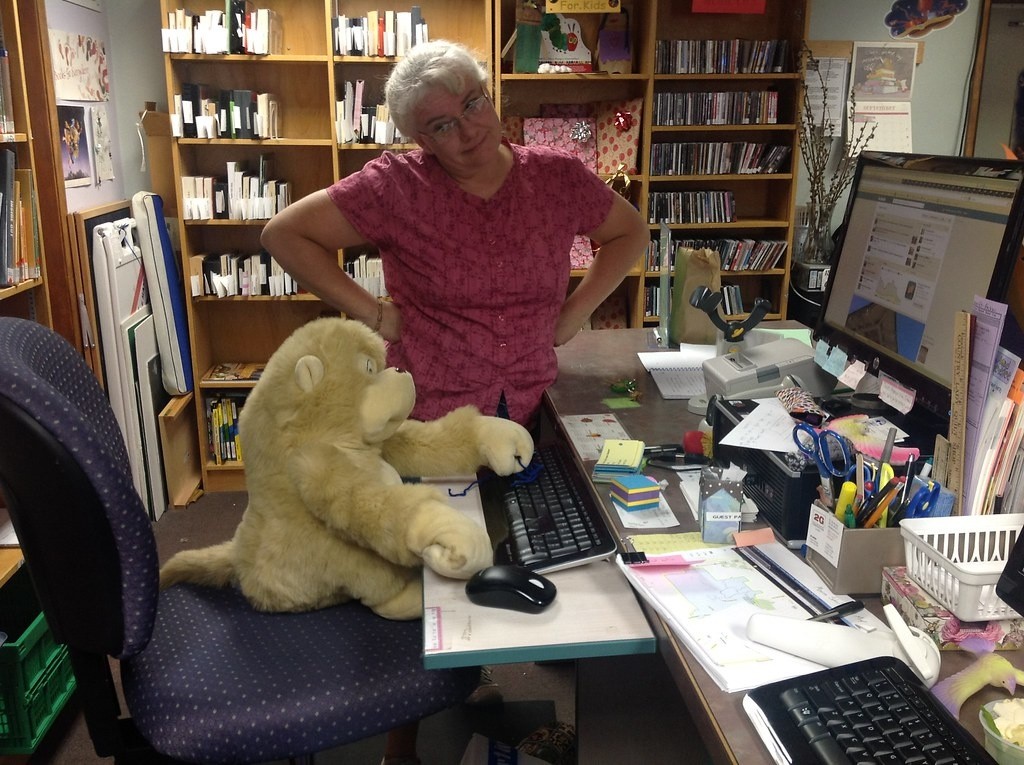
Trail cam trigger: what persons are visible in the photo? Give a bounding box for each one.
[261,38,649,704]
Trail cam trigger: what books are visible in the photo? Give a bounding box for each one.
[335,79,412,144]
[204,362,266,465]
[655,39,786,73]
[331,6,428,57]
[652,92,777,125]
[189,252,389,297]
[650,142,788,176]
[637,352,706,399]
[646,238,787,272]
[161,0,278,54]
[171,84,282,138]
[182,155,291,220]
[646,285,743,317]
[648,191,733,224]
[0,50,41,284]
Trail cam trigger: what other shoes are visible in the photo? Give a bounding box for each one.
[463,666,502,706]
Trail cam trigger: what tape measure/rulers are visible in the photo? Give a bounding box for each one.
[946,311,970,516]
[932,433,949,488]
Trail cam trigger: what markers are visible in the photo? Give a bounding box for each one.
[834,481,856,523]
[643,442,684,456]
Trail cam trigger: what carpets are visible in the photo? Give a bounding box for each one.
[313,699,557,765]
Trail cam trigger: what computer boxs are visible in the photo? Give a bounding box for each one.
[712,398,951,545]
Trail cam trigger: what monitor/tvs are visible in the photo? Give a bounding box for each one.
[809,150,1024,413]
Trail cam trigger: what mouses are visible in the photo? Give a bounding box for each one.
[465,564,557,614]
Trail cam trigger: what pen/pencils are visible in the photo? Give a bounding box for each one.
[844,503,856,529]
[856,477,899,525]
[864,476,906,528]
[888,498,911,527]
[901,454,915,505]
[807,600,867,623]
[919,457,934,477]
[876,427,896,479]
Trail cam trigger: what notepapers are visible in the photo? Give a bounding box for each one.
[609,475,661,512]
[592,440,646,482]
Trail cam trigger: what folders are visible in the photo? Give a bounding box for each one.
[182,83,200,138]
[219,88,254,139]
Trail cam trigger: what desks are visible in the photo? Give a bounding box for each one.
[546,320,1024,765]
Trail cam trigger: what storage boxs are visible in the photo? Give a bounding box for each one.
[805,497,1024,652]
[697,465,743,544]
[0,610,77,758]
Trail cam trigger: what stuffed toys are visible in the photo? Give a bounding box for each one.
[158,317,535,620]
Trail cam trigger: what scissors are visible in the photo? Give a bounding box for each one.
[906,481,941,518]
[845,464,897,508]
[792,423,852,512]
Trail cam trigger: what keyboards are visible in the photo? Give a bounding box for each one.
[742,654,1000,765]
[477,440,616,575]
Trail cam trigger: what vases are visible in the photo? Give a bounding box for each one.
[799,200,836,271]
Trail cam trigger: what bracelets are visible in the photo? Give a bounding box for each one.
[374,299,382,331]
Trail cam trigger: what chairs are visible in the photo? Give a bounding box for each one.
[0,316,485,765]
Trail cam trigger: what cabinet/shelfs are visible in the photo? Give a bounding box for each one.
[159,0,495,496]
[0,0,60,588]
[493,0,812,328]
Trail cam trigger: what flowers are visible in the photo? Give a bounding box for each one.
[794,40,878,263]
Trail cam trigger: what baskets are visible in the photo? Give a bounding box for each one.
[899,513,1023,622]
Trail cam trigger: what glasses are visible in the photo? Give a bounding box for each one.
[418,89,490,148]
